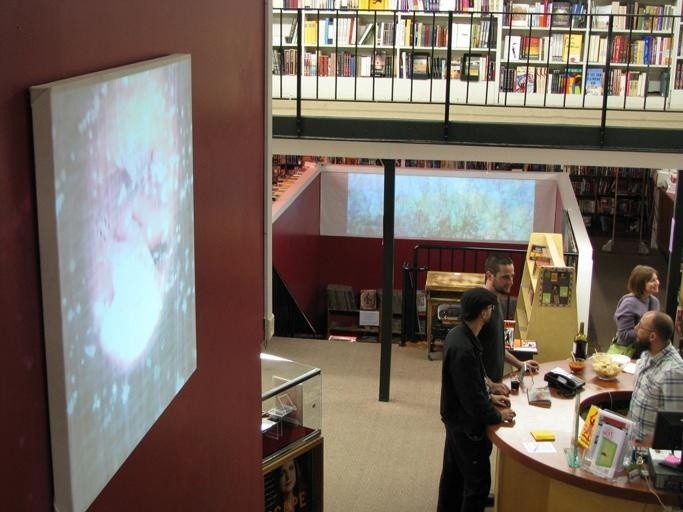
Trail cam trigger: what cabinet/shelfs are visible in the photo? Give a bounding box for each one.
[514,232,579,361]
[272,0,683,113]
[328,299,425,337]
[321,153,553,172]
[553,164,650,262]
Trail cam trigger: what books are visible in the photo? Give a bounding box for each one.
[272,153,316,204]
[272,1,506,27]
[527,387,552,405]
[585,409,635,482]
[285,13,492,48]
[272,375,304,426]
[675,31,682,90]
[272,47,496,83]
[316,156,570,173]
[570,165,679,240]
[503,31,674,66]
[325,245,571,354]
[508,1,677,32]
[498,65,670,97]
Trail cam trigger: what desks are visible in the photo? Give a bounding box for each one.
[425,271,485,361]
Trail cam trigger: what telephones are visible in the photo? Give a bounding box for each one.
[544,367,586,392]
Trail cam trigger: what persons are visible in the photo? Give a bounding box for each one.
[623,310,682,446]
[437,287,516,512]
[474,254,540,507]
[612,264,660,358]
[273,461,304,512]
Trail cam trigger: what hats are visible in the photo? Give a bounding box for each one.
[460,287,498,309]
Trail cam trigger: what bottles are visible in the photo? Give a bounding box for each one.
[572,322,589,360]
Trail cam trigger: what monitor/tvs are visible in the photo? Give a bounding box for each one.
[652,411,683,472]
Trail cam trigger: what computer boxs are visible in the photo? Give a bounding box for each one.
[647,447,683,491]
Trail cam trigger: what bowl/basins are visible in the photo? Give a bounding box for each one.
[567,358,586,373]
[588,353,630,383]
